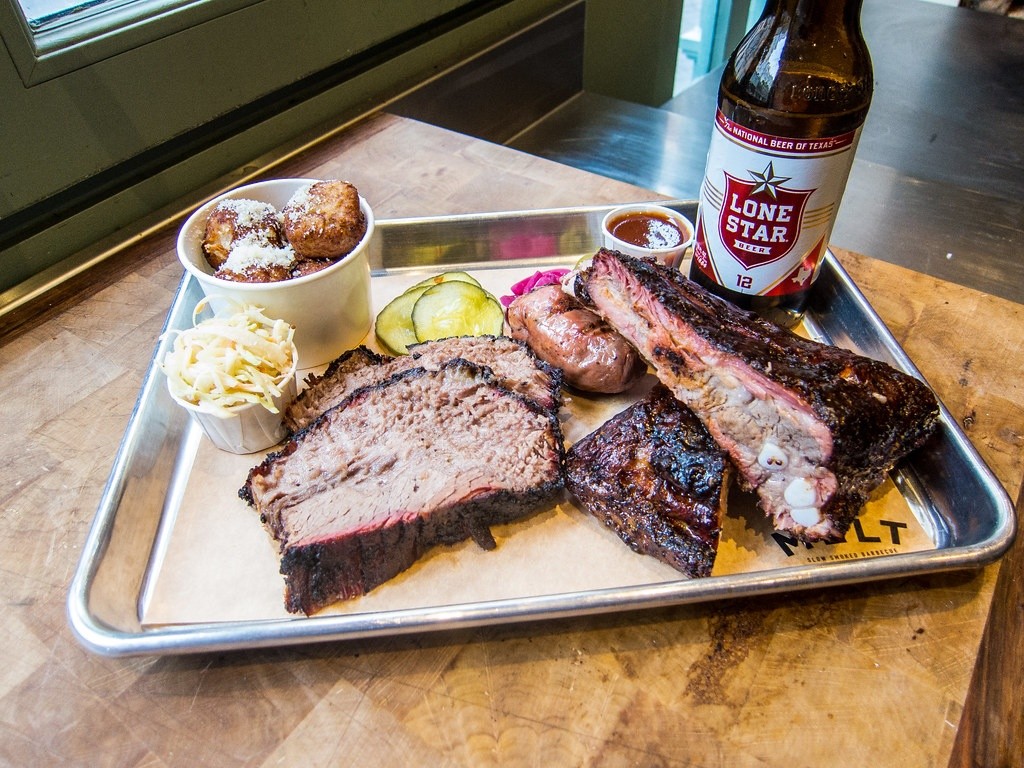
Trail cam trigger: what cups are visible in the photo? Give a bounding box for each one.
[597,203,696,270]
[167,342,299,454]
[176,179,376,371]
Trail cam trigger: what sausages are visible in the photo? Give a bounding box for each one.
[503,285,650,394]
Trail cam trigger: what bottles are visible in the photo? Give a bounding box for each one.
[690,1,873,330]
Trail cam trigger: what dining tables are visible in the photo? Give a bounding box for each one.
[0,113,1024,768]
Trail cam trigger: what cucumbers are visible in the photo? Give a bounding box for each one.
[373,270,505,357]
